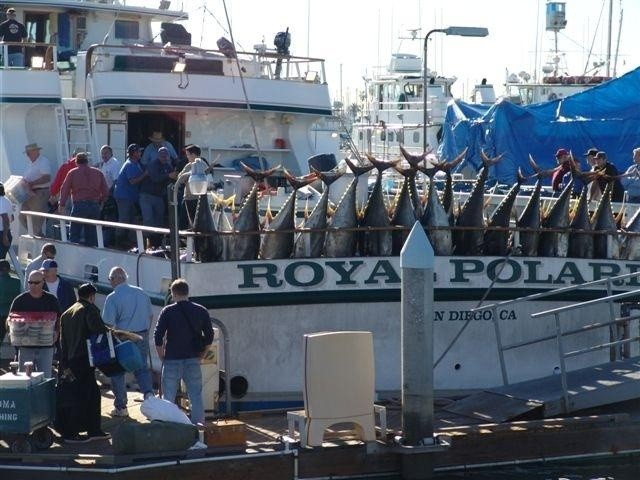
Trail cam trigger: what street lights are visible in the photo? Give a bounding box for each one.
[421,25,489,186]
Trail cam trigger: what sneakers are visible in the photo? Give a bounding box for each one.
[110,407,128,416]
[64,433,92,443]
[89,431,111,439]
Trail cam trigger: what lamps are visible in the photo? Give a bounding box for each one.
[171,61,187,74]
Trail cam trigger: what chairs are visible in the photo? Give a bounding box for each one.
[285,328,388,449]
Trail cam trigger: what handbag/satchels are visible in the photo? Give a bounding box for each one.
[192,331,205,352]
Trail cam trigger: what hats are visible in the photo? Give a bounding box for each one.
[158,146,168,156]
[148,132,165,142]
[78,283,97,297]
[593,151,606,159]
[556,149,568,155]
[23,143,42,152]
[127,143,144,151]
[584,148,598,156]
[39,259,57,270]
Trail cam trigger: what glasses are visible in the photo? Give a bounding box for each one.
[45,254,55,258]
[28,280,41,284]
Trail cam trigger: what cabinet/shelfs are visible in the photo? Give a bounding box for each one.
[202,145,292,171]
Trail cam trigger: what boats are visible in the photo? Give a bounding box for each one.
[332,52,497,181]
[0,0,640,415]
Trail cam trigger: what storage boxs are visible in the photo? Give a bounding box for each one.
[205,419,246,449]
[7,310,59,348]
[2,174,38,207]
[222,174,254,205]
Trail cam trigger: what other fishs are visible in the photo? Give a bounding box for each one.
[591,172,627,258]
[432,145,471,256]
[227,158,278,261]
[570,166,606,257]
[292,169,347,259]
[324,157,376,257]
[210,189,236,261]
[182,155,227,261]
[358,153,402,257]
[484,166,543,256]
[538,149,593,256]
[453,150,508,256]
[415,159,454,256]
[261,169,322,259]
[510,152,564,256]
[390,162,425,256]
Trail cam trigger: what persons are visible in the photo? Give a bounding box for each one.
[102,266,154,416]
[0,183,15,259]
[0,261,21,346]
[38,259,77,312]
[5,270,62,379]
[550,147,640,204]
[24,244,57,290]
[0,8,29,67]
[60,283,114,443]
[18,128,223,263]
[153,277,214,425]
